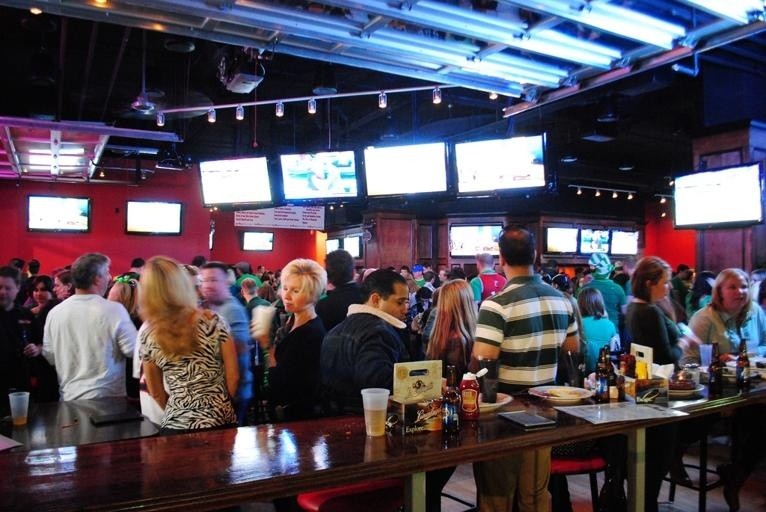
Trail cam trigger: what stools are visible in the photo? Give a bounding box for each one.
[548,454,607,512]
[295,475,405,512]
[661,425,732,511]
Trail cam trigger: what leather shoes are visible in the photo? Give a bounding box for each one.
[719,463,741,511]
[664,447,693,486]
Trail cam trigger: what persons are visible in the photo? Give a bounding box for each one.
[2,223,765,510]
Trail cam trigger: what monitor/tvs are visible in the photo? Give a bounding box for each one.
[125,200,183,236]
[449,130,558,200]
[240,230,274,251]
[325,235,362,259]
[672,159,766,230]
[24,193,92,233]
[544,225,640,255]
[449,223,504,258]
[197,151,281,211]
[360,138,452,203]
[272,143,363,207]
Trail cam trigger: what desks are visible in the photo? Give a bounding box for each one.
[1,396,160,455]
[1,363,766,512]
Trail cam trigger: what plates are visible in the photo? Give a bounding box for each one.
[466,392,513,415]
[669,359,766,396]
[529,385,595,405]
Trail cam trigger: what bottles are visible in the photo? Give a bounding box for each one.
[709,339,724,397]
[459,368,490,422]
[440,363,462,448]
[736,338,752,395]
[620,354,635,379]
[595,343,616,402]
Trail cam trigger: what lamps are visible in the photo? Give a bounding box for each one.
[566,184,638,201]
[155,83,498,128]
[654,193,673,203]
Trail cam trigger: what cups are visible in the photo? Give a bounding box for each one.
[8,392,29,425]
[361,386,390,436]
[478,356,501,402]
[253,306,277,335]
